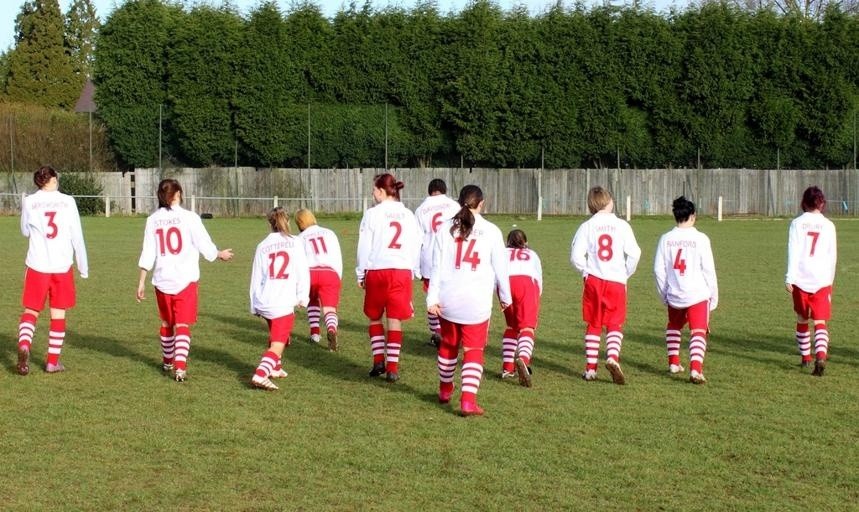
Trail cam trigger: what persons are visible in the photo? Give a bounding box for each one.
[494,229,543,386]
[293,207,343,351]
[652,196,719,385]
[134,179,235,382]
[571,185,642,383]
[250,206,311,389]
[784,185,838,377]
[354,173,422,383]
[424,184,514,415]
[17,165,89,375]
[415,178,462,346]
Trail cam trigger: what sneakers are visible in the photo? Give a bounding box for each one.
[44,357,66,374]
[439,385,456,404]
[269,366,290,380]
[800,360,813,370]
[251,374,279,391]
[368,359,387,378]
[386,369,402,384]
[429,335,441,346]
[604,356,626,386]
[581,367,598,382]
[162,361,175,372]
[668,362,686,375]
[460,399,486,416]
[15,341,33,377]
[689,369,709,386]
[172,368,187,383]
[515,357,533,388]
[501,367,516,380]
[326,328,340,353]
[310,333,322,345]
[811,358,826,376]
